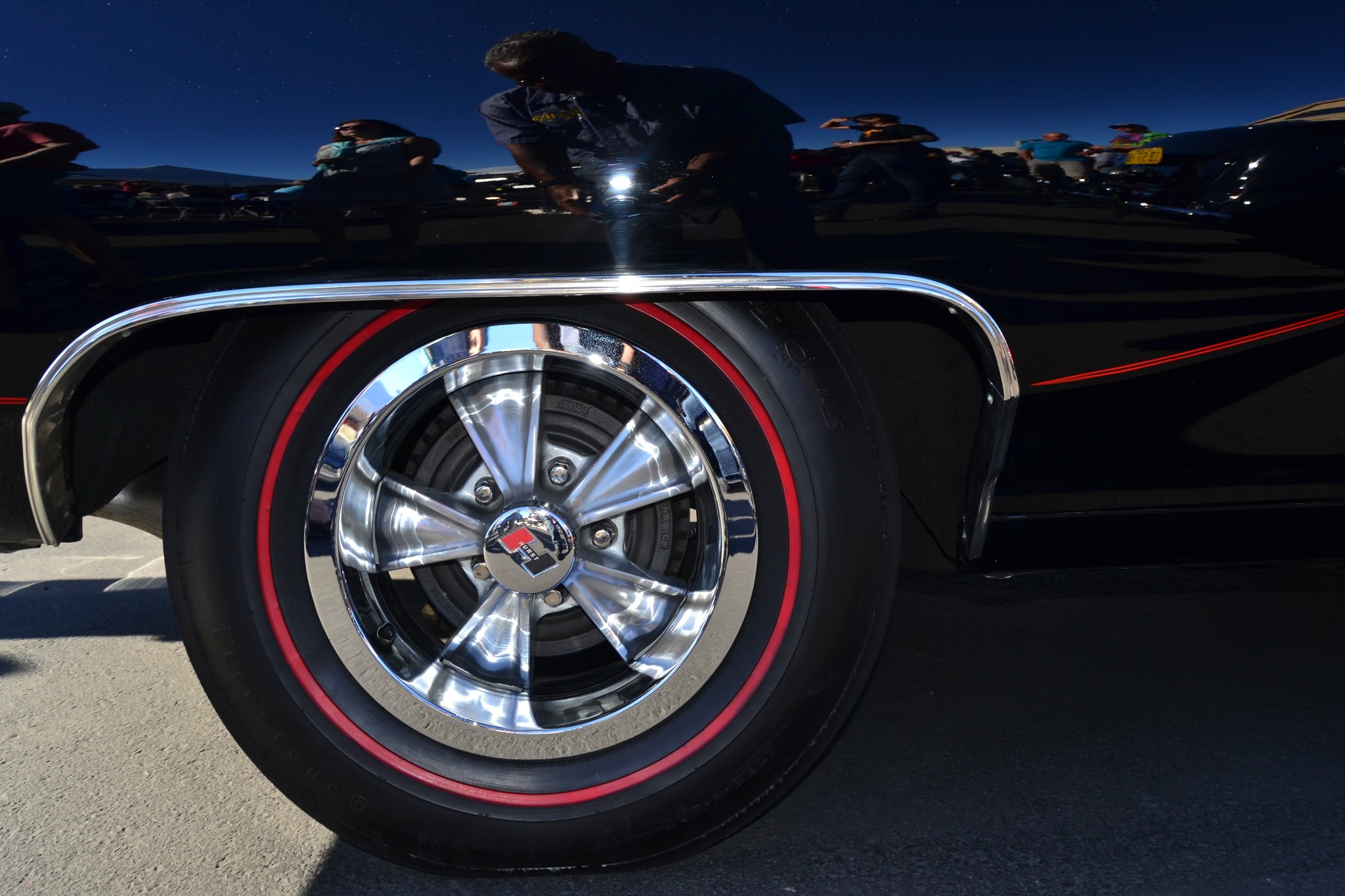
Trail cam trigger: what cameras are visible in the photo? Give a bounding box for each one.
[588,157,668,210]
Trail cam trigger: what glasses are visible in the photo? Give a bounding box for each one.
[516,64,550,87]
[335,122,374,133]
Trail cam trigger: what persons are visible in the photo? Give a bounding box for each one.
[482,30,807,244]
[809,114,1170,228]
[0,103,101,191]
[118,119,495,221]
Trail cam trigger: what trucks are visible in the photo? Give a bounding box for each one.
[1,0,1345,881]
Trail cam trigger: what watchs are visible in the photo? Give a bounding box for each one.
[532,175,575,190]
